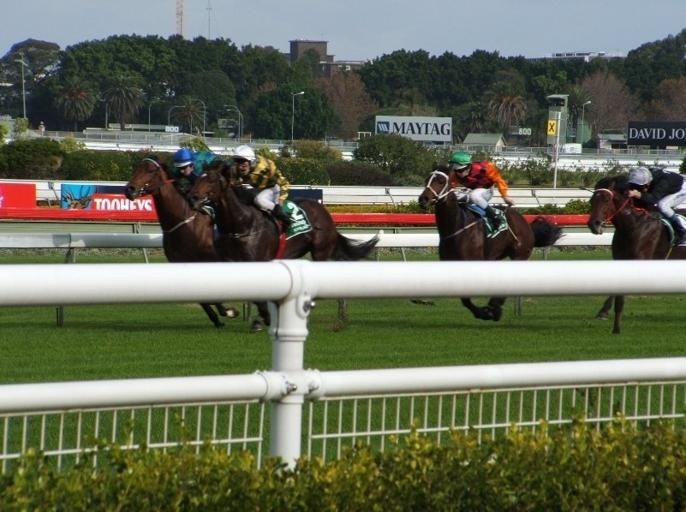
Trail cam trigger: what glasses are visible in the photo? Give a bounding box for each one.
[454,168,468,173]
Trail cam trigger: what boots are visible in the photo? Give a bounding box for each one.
[668,213,685,247]
[271,207,291,234]
[484,206,506,233]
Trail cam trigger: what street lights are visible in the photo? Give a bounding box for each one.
[581,100,592,143]
[291,91,305,140]
[224,104,244,138]
[192,98,206,137]
[167,104,185,125]
[148,98,165,132]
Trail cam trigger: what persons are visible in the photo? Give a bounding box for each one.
[627,165,686,247]
[171,147,226,243]
[230,144,294,234]
[448,150,516,239]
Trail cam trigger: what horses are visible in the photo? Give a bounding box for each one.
[124,155,240,329]
[418,162,567,321]
[186,158,380,331]
[588,174,686,334]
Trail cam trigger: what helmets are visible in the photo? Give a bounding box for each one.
[231,145,255,163]
[627,166,653,186]
[173,149,194,168]
[449,151,472,165]
